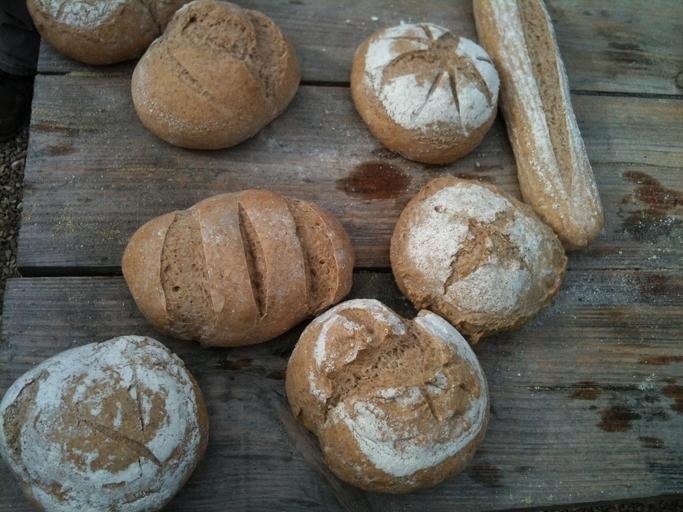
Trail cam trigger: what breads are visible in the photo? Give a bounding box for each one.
[0,334,209,512]
[390,173,568,348]
[25,0,190,66]
[121,188,355,348]
[473,0,606,253]
[350,22,501,166]
[131,0,300,150]
[284,299,491,494]
